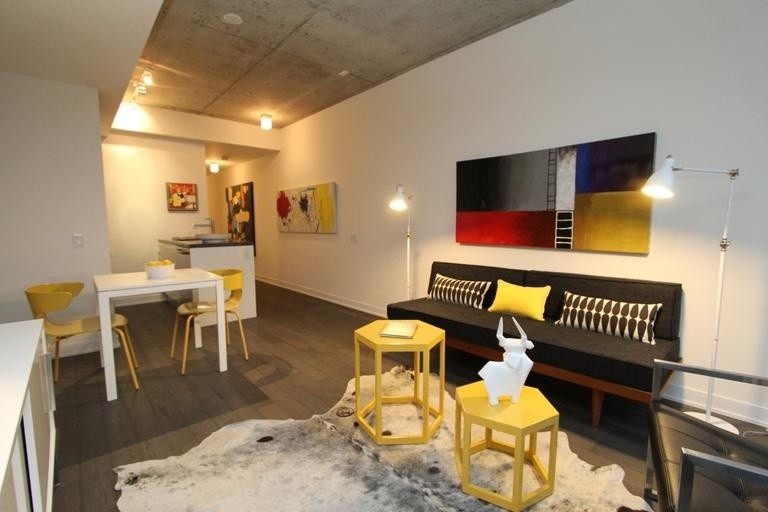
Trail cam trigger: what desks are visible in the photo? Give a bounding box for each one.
[92,267,228,400]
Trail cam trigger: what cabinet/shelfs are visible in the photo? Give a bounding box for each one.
[1,319,56,511]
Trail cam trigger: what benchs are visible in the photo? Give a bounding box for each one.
[388,261,681,429]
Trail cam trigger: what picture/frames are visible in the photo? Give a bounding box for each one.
[167,182,198,211]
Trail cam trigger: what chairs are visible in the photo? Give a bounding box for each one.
[646,361,768,510]
[26,268,141,389]
[170,270,250,375]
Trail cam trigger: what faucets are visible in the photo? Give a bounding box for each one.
[193,216,215,233]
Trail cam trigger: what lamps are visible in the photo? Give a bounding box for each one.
[638,155,741,436]
[389,183,409,302]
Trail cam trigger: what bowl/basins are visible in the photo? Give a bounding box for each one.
[144,262,175,280]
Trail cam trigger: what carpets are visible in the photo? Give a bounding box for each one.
[115,365,659,512]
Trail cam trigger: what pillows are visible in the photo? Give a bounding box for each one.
[426,273,664,346]
